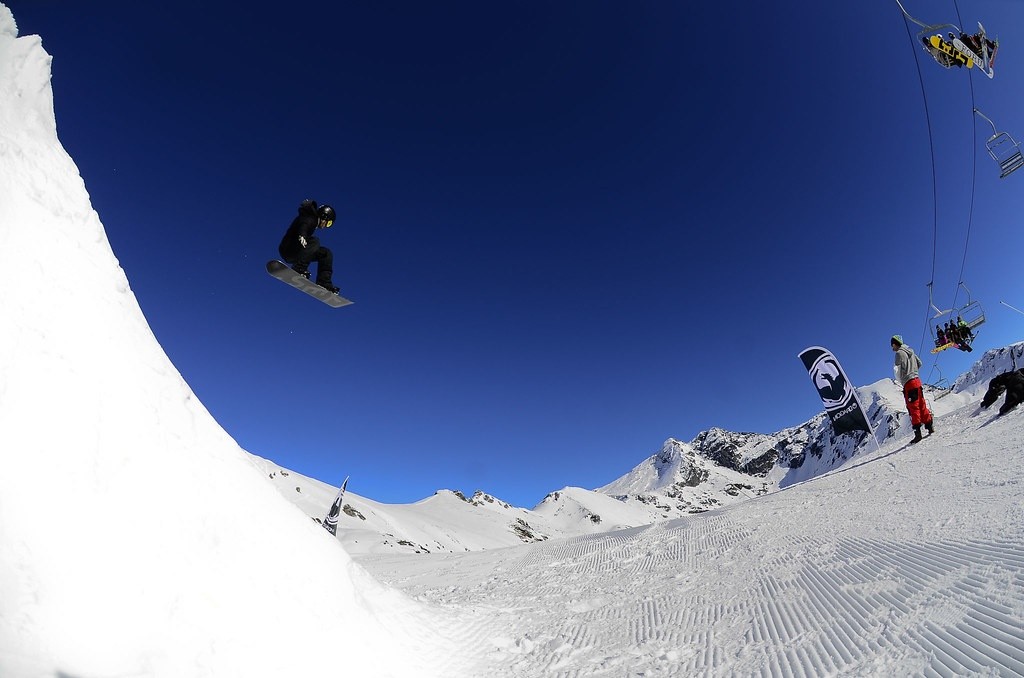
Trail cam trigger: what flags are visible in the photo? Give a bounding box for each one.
[800,349,874,438]
[321,479,348,539]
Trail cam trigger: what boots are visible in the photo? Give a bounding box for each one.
[910,430,922,444]
[928,426,934,434]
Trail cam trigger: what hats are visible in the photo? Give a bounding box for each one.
[950,320,954,324]
[936,325,940,329]
[891,335,903,347]
[958,316,962,321]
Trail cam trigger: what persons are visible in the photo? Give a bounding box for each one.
[944,323,954,343]
[890,335,934,444]
[957,317,975,339]
[936,325,946,351]
[279,198,340,295]
[922,32,995,68]
[950,319,960,340]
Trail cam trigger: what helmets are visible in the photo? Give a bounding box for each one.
[318,205,336,229]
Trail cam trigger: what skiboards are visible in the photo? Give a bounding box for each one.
[962,337,970,345]
[970,330,979,347]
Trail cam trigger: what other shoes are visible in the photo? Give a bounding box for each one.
[331,287,340,294]
[301,270,311,279]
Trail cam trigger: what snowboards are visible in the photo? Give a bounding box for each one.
[265,258,354,309]
[930,341,954,354]
[950,341,966,352]
[929,21,999,79]
[957,337,972,353]
